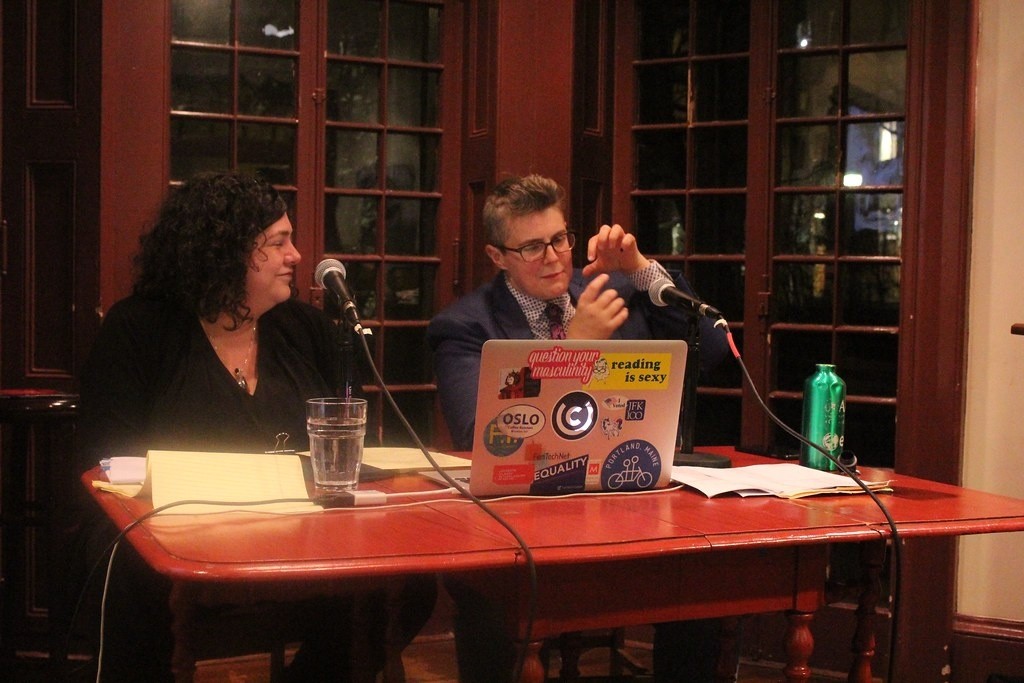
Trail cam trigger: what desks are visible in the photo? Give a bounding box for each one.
[82,440,1023,683]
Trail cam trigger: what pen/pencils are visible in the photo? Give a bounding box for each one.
[98,466,110,473]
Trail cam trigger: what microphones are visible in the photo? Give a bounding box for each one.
[648,277,721,321]
[315,260,361,328]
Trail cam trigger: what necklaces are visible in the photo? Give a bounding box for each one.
[200,312,256,391]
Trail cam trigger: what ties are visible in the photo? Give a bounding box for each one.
[545,304,567,340]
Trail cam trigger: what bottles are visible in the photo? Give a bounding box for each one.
[798,363,848,471]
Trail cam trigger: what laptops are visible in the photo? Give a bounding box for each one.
[419,340,688,498]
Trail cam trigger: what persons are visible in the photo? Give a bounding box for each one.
[425,172,734,683]
[73,169,437,683]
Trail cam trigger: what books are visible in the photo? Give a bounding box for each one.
[294,447,474,484]
[671,461,895,499]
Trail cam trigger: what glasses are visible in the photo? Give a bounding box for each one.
[499,231,576,262]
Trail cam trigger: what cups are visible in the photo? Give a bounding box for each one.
[305,397,368,492]
